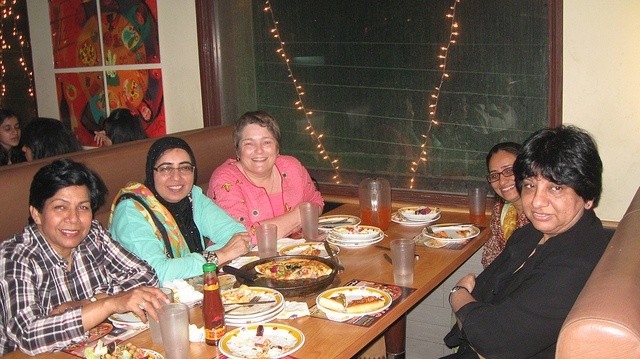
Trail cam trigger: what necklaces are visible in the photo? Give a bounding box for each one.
[62,251,73,265]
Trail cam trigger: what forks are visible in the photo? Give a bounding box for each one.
[225,296,261,315]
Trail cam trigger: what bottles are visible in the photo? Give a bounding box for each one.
[202,263,226,345]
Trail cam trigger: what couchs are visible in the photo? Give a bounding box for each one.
[0,125,237,241]
[0,126,220,171]
[555,186,639,358]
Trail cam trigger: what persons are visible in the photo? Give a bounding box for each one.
[18,116,84,162]
[93,107,147,148]
[362,94,428,173]
[456,68,490,127]
[0,158,170,359]
[206,110,325,248]
[108,136,252,287]
[430,88,491,176]
[0,109,27,166]
[481,141,531,270]
[438,124,611,359]
[476,75,522,145]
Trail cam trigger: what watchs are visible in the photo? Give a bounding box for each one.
[90,296,97,303]
[451,287,457,293]
[207,252,217,262]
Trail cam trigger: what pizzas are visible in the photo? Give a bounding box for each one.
[221,290,249,305]
[346,295,386,313]
[254,257,333,281]
[318,292,346,314]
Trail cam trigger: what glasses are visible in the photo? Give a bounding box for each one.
[153,166,195,175]
[486,167,514,183]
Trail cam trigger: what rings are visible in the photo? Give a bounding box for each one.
[139,300,148,308]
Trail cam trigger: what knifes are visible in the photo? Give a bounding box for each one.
[324,241,340,272]
[375,245,391,251]
[384,253,392,264]
[224,300,276,307]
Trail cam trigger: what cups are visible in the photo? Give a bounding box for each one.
[255,224,277,261]
[147,287,176,345]
[299,201,319,240]
[389,238,415,288]
[467,187,487,224]
[159,303,191,359]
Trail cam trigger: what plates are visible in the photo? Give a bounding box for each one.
[318,215,361,230]
[398,206,440,220]
[224,309,288,326]
[108,310,148,328]
[220,286,287,319]
[326,237,385,249]
[391,213,440,227]
[222,255,342,295]
[327,233,384,243]
[316,286,392,314]
[423,222,480,240]
[279,241,340,258]
[94,347,165,359]
[218,323,305,359]
[223,299,286,322]
